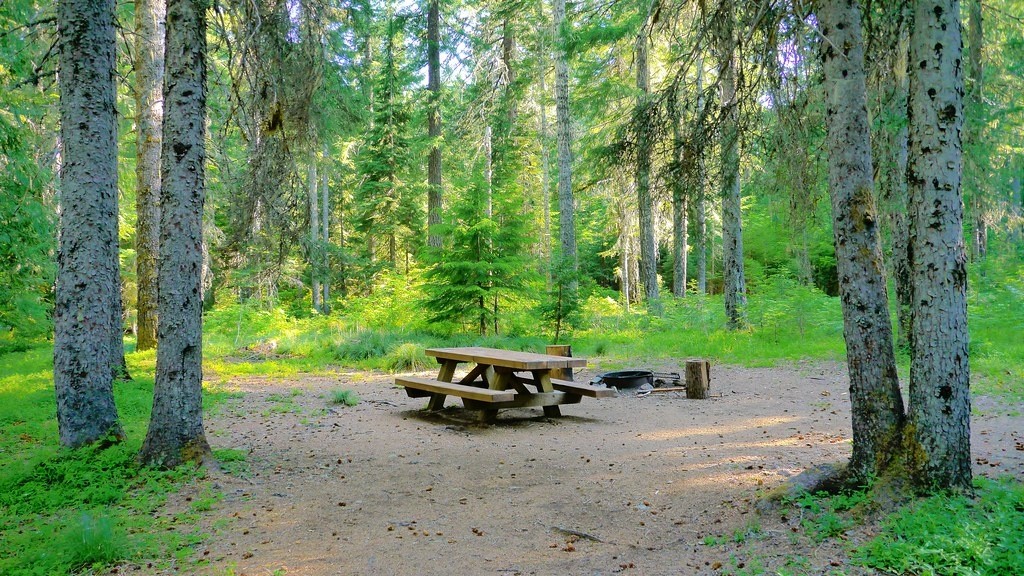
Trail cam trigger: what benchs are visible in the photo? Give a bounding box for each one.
[395,377,514,402]
[517,375,614,398]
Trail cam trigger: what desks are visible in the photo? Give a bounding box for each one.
[425,346,587,418]
